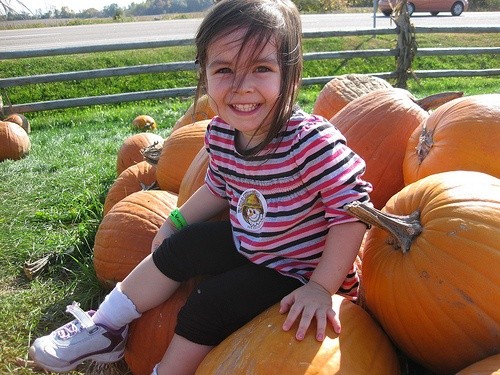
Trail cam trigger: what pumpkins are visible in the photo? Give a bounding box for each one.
[117,132,165,176]
[141,93,230,222]
[0,120,31,162]
[448,353,500,375]
[93,189,179,289]
[312,73,394,121]
[328,87,464,210]
[123,276,195,375]
[192,294,405,375]
[402,93,500,187]
[104,160,156,219]
[132,115,156,132]
[3,113,31,133]
[344,171,500,371]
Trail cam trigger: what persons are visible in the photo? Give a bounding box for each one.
[31,0,375,375]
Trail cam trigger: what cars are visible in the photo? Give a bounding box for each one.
[378,0,470,18]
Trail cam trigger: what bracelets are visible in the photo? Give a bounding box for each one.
[168,208,189,230]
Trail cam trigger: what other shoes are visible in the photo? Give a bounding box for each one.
[29,301,129,373]
